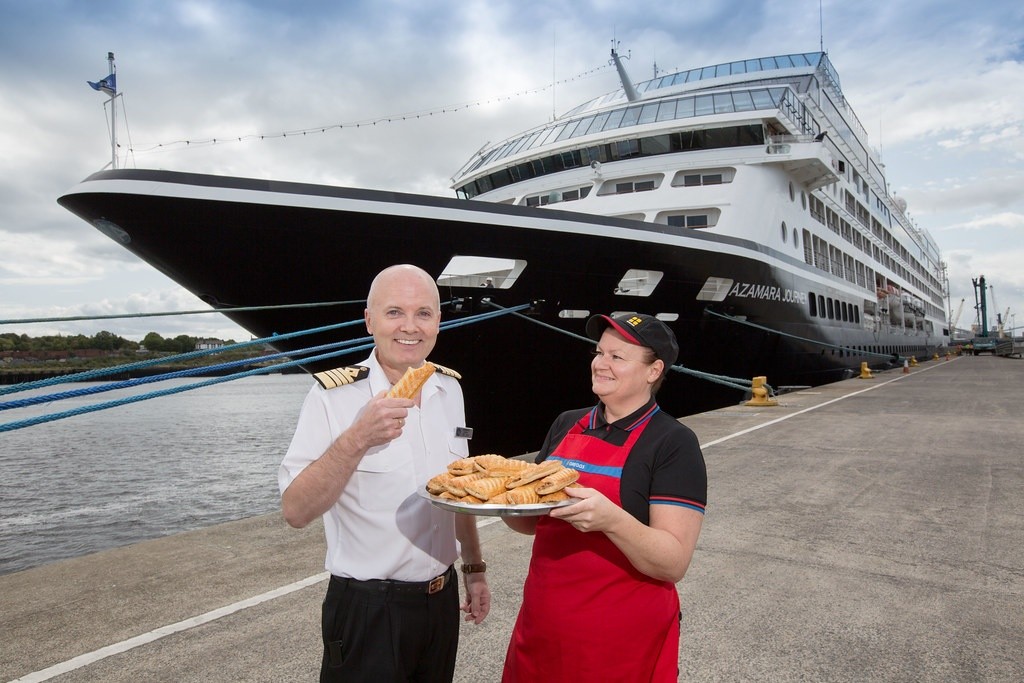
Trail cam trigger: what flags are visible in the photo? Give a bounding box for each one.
[87,73,116,96]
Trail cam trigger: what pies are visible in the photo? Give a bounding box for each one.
[426,454,584,503]
[385,364,435,398]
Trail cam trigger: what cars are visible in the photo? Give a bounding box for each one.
[973,336,997,355]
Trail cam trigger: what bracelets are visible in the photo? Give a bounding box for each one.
[461,559,486,573]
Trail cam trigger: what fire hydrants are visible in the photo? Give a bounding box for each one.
[945,351,950,361]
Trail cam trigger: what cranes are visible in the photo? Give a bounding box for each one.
[990,285,1003,338]
[1002,307,1010,328]
[952,299,965,331]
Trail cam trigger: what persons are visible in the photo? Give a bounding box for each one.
[966,343,973,355]
[957,344,962,355]
[502,315,708,683]
[277,264,491,683]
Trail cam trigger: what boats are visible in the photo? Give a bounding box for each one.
[56,51,950,457]
[876,285,900,309]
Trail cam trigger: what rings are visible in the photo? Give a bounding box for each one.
[397,418,401,428]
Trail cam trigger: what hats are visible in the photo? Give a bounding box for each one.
[586,312,679,375]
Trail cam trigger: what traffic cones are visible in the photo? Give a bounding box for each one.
[903,359,909,374]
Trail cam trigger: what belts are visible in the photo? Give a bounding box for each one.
[334,565,454,596]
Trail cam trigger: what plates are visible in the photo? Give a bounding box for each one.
[418,480,583,517]
